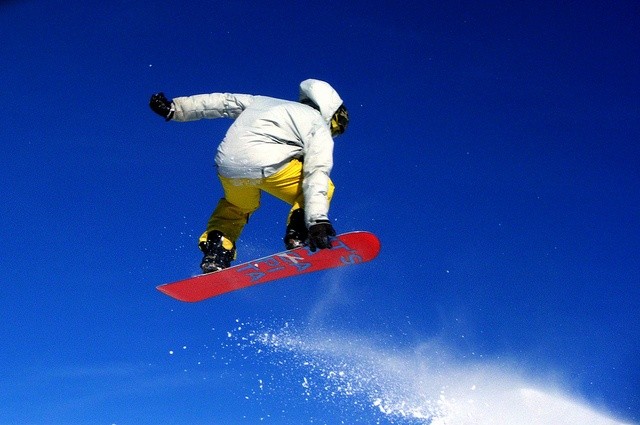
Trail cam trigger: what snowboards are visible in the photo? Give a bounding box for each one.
[155,231,381,302]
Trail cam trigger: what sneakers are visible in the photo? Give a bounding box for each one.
[199,241,234,274]
[285,221,308,249]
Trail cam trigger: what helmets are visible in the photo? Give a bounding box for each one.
[332,106,348,131]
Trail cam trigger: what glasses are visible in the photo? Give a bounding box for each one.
[331,119,340,138]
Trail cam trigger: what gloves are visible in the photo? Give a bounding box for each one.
[149,92,172,117]
[309,224,335,252]
